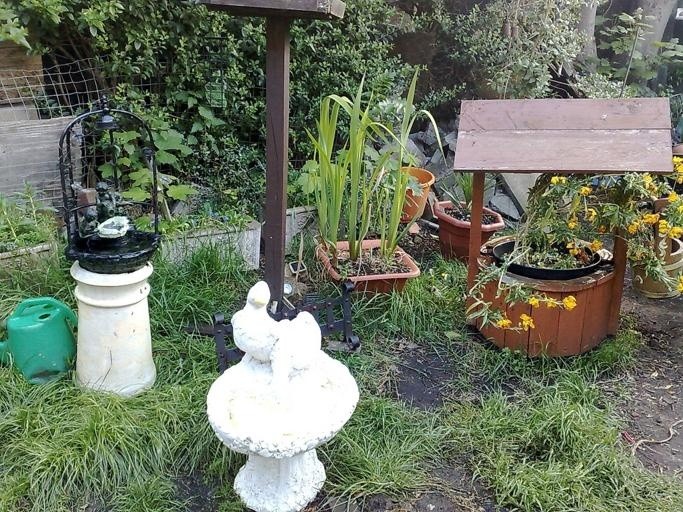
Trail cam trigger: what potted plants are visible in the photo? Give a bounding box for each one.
[307,71,421,296]
[435,170,502,257]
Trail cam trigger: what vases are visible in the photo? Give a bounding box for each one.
[382,166,433,218]
[477,237,621,359]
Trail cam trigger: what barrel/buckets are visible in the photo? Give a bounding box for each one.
[399,166,435,218]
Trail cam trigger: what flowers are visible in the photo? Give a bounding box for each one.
[461,158,682,339]
